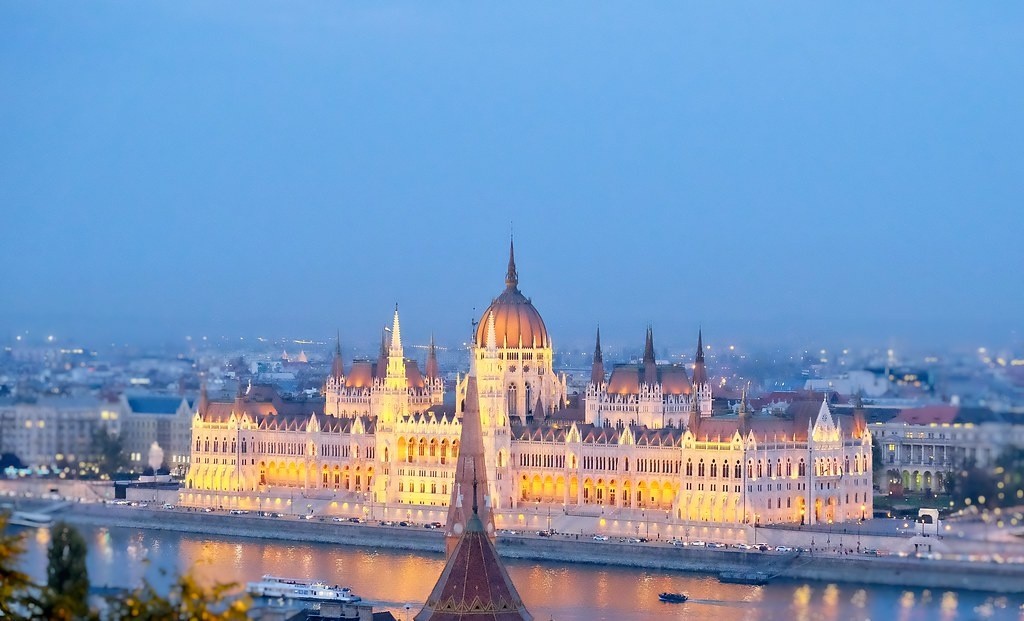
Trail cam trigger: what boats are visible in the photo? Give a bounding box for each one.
[246,573,363,606]
